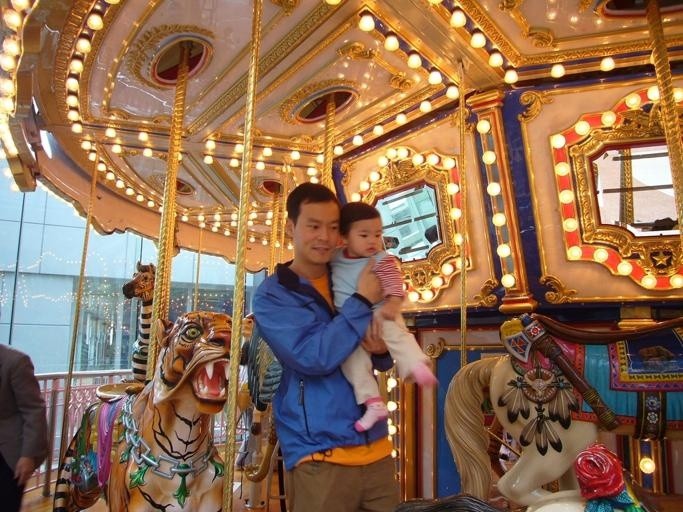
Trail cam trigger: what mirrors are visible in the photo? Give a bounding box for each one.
[565,106,683,265]
[359,161,456,288]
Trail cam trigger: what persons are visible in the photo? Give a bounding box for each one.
[331,205,437,434]
[1,343,50,512]
[254,182,401,512]
